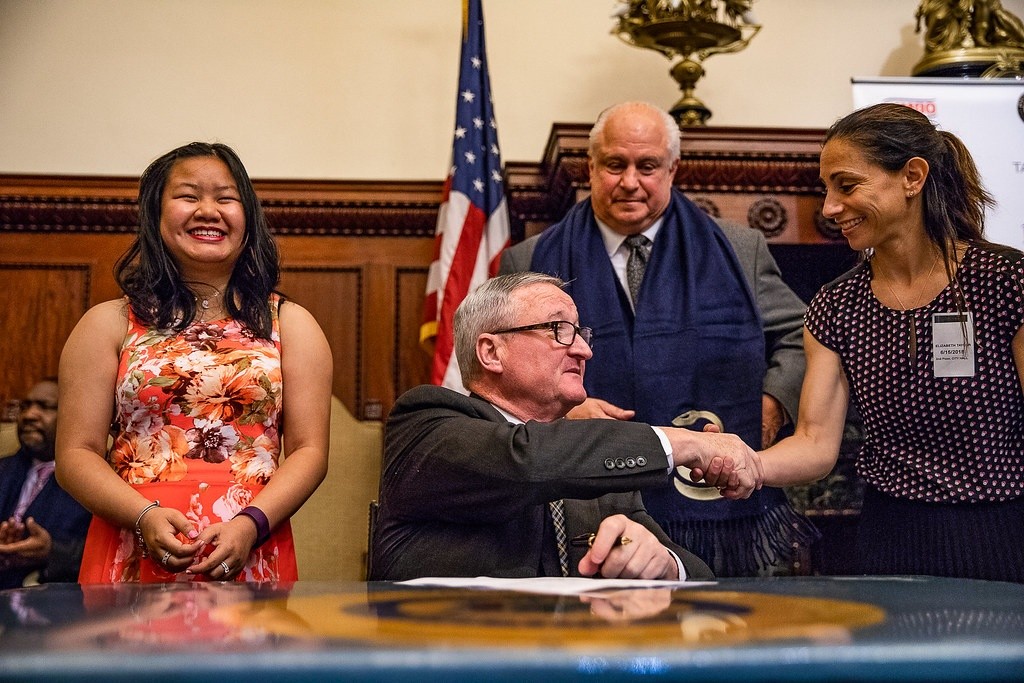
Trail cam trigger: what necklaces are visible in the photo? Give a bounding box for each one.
[193,284,228,309]
[874,254,940,375]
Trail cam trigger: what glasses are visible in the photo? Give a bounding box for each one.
[491,320,596,352]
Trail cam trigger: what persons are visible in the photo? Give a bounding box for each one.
[375,271,766,580]
[55,142,333,593]
[497,101,812,581]
[0,376,93,589]
[690,104,1024,583]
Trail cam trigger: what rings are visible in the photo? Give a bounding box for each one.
[715,485,727,490]
[161,551,172,566]
[220,561,229,574]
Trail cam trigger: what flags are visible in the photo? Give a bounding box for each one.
[419,0,512,402]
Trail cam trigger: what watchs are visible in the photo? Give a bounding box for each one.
[231,506,270,551]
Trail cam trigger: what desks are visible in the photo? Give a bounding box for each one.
[0,574,1024,683]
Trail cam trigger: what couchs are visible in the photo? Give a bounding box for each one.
[0,395,382,586]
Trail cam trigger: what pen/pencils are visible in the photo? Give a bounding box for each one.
[569,532,634,548]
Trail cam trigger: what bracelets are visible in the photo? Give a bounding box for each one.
[134,500,162,559]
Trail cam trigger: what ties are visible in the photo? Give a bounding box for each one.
[622,233,651,316]
[549,498,570,575]
[28,464,54,504]
